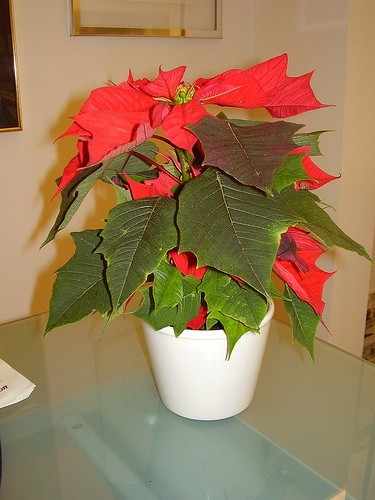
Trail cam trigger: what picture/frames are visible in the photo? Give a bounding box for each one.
[0,0,22,134]
[72,1,221,40]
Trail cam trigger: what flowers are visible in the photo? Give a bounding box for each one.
[42,53,370,361]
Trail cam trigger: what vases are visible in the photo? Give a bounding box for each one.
[137,298,274,421]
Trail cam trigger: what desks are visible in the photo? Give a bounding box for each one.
[0,293,373,500]
